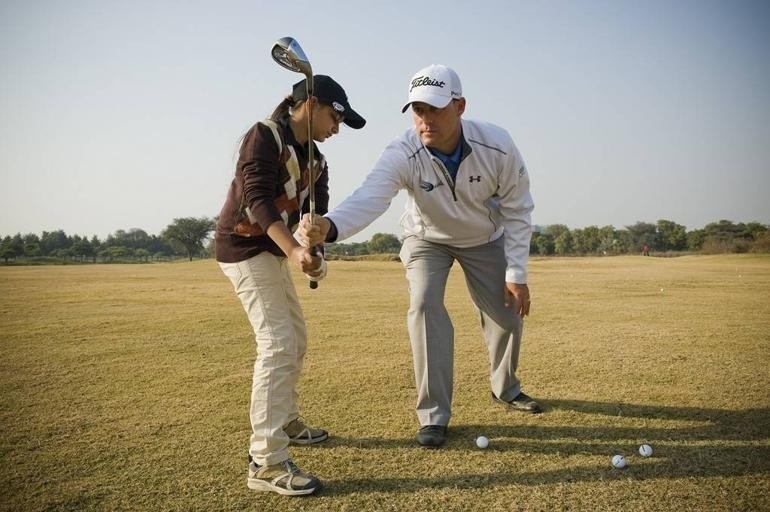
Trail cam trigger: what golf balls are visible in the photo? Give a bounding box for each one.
[475,436,489,449]
[638,444,653,457]
[611,454,627,470]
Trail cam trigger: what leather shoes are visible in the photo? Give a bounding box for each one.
[491,391,540,412]
[417,426,447,446]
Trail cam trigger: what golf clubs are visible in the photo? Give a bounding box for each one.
[271,36,320,289]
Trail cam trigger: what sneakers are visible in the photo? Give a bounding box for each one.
[284,418,328,445]
[247,455,323,496]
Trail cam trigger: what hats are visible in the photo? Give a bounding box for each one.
[293,75,366,129]
[401,64,463,113]
